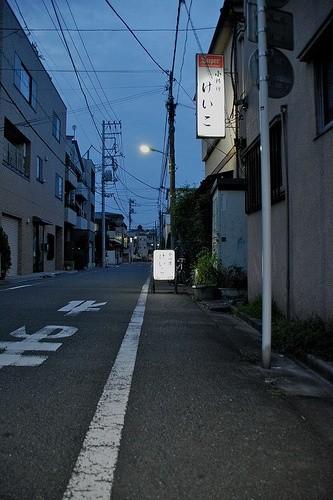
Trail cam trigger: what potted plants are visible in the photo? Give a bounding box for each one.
[219,264,248,301]
[192,249,220,300]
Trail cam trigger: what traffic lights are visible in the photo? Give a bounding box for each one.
[129,199,135,214]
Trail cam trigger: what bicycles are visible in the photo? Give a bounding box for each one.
[168,258,194,288]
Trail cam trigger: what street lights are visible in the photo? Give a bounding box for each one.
[141,145,176,251]
